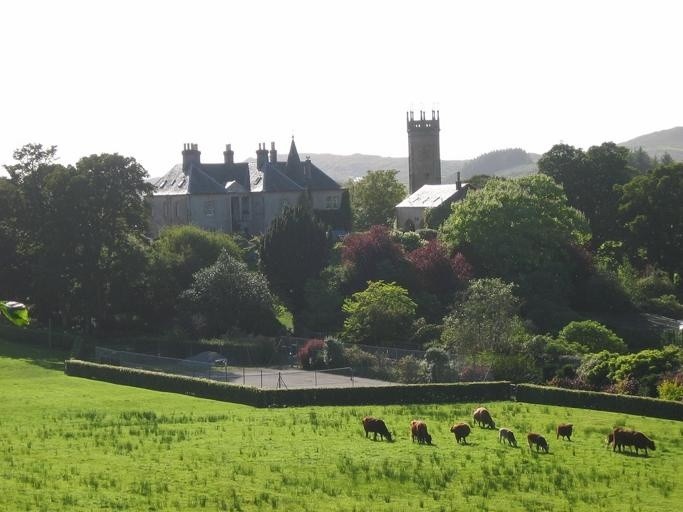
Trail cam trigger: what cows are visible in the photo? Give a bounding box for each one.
[611,425,656,457]
[556,422,573,442]
[606,432,633,452]
[527,432,550,454]
[473,407,496,430]
[449,423,471,444]
[409,420,433,446]
[361,415,392,442]
[499,427,519,448]
